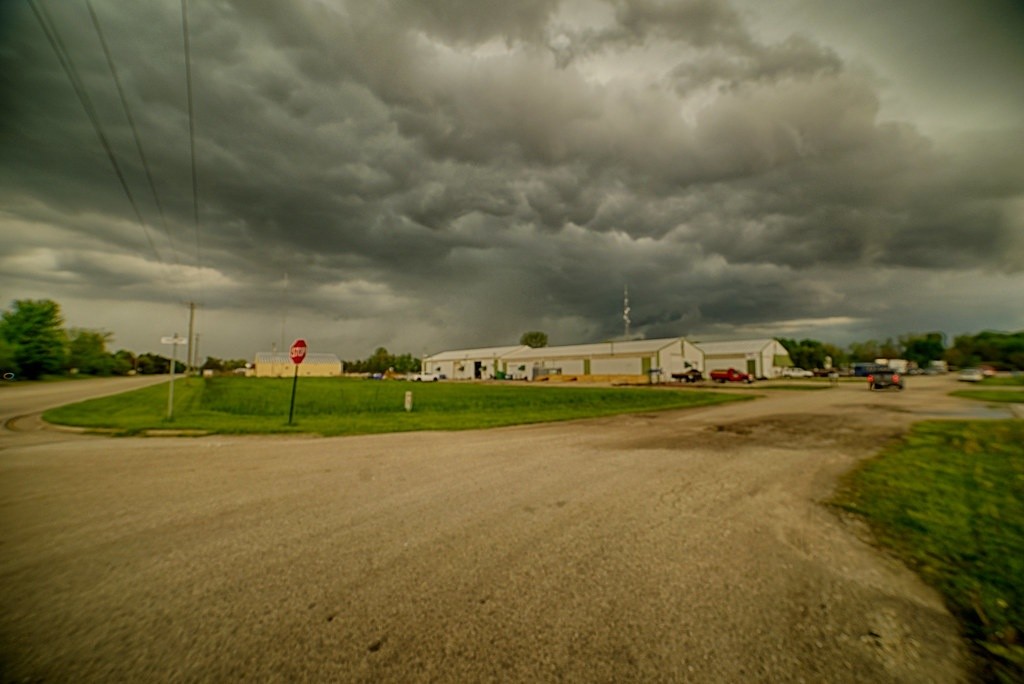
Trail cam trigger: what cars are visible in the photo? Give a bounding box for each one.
[956,362,996,383]
[781,361,946,377]
[867,368,904,390]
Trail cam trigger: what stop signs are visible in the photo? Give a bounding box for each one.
[290,338,308,364]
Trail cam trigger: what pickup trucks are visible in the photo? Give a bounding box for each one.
[709,368,752,384]
